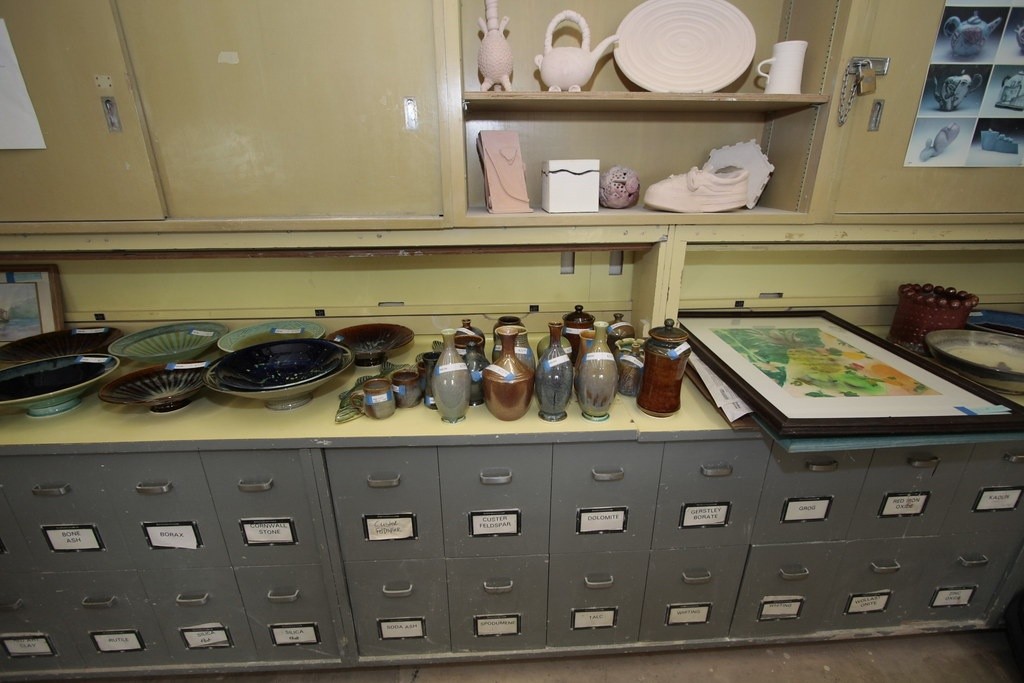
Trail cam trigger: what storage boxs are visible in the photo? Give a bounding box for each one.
[540,160,600,212]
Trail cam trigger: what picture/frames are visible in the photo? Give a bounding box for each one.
[0,264,65,345]
[678,307,1024,440]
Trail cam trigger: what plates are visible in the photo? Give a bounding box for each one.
[0,326,119,362]
[613,0,756,93]
[925,329,1024,393]
[967,308,1024,337]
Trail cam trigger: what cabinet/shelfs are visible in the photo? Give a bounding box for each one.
[459,0,868,244]
[0,0,469,252]
[823,0,1024,243]
[0,335,350,683]
[313,334,775,666]
[727,325,1024,645]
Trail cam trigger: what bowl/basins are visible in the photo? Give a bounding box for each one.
[109,321,222,360]
[97,359,208,414]
[0,354,119,418]
[218,319,327,353]
[325,322,415,368]
[202,337,353,412]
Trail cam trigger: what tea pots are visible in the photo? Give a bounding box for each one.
[932,69,983,111]
[534,10,620,92]
[943,11,1002,57]
[995,70,1024,109]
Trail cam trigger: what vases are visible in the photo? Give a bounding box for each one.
[534,322,573,422]
[431,328,472,423]
[576,320,620,420]
[482,326,536,422]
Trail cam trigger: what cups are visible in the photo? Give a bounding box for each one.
[614,337,645,363]
[349,379,394,420]
[561,304,596,363]
[757,40,808,94]
[386,366,423,408]
[415,351,442,410]
[453,335,484,357]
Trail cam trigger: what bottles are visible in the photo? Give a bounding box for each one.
[636,318,690,418]
[574,322,618,422]
[482,327,534,422]
[492,326,536,370]
[537,319,572,422]
[574,328,608,374]
[620,344,644,396]
[606,313,636,355]
[455,318,485,350]
[490,310,525,340]
[432,327,471,423]
